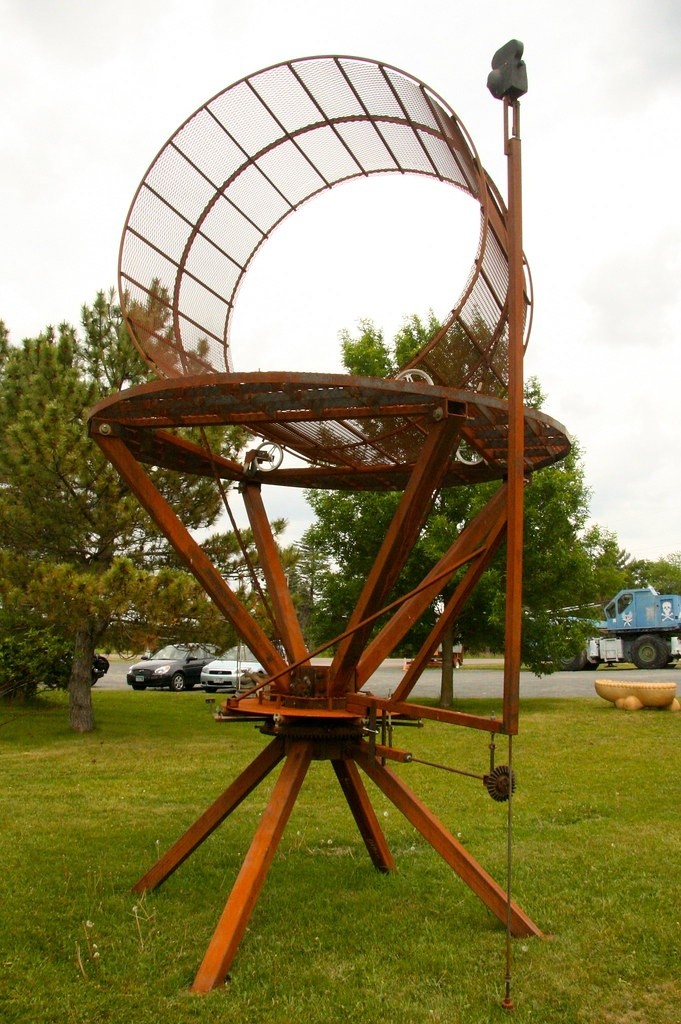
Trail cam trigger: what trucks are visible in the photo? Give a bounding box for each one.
[403,639,463,672]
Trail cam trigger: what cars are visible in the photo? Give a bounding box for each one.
[201,640,309,693]
[127,643,226,691]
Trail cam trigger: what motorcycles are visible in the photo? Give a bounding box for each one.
[36,649,109,688]
